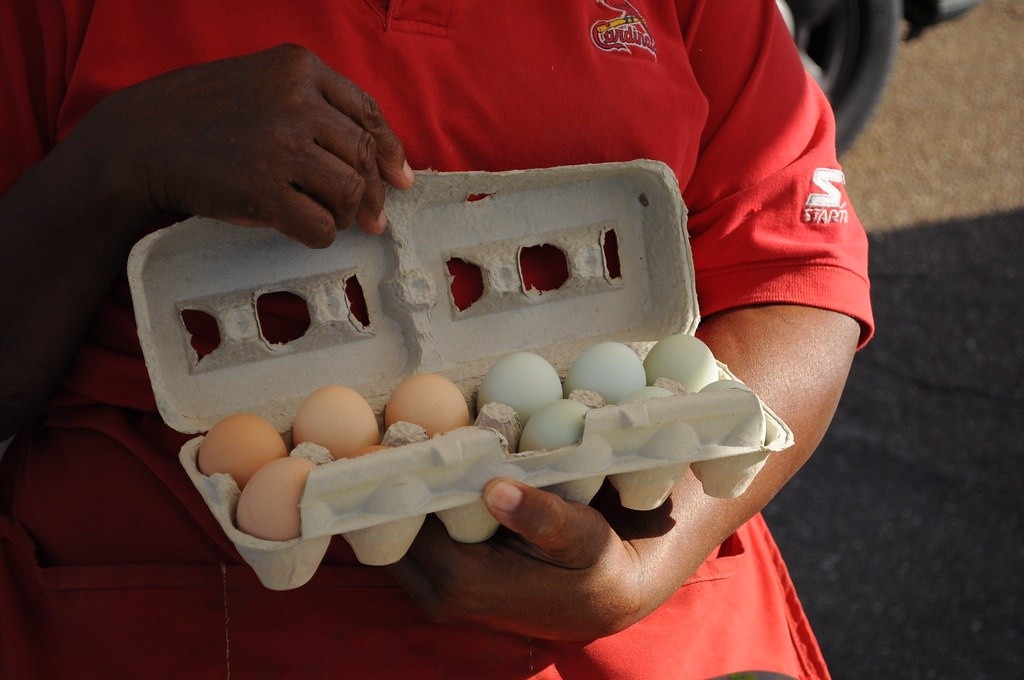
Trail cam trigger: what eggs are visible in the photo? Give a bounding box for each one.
[199,332,768,540]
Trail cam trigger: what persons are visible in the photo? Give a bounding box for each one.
[0,2,874,679]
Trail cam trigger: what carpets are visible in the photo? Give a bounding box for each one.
[837,0,1024,232]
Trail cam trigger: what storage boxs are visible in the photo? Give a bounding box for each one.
[127,159,796,591]
